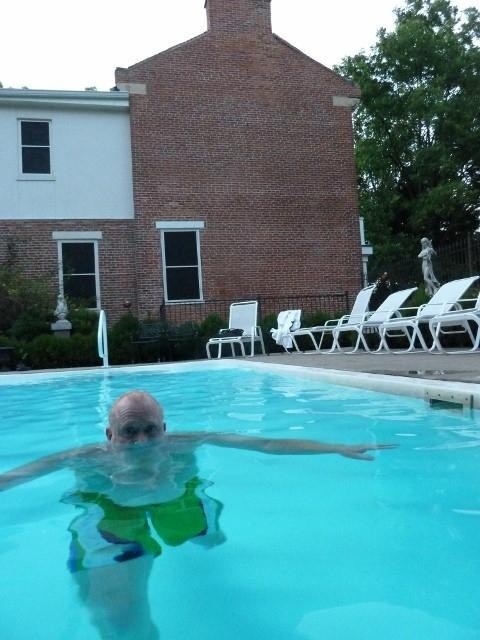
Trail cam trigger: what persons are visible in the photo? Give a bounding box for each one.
[1,390,409,640]
[417,237,441,296]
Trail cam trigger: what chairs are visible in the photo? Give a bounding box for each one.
[206,272,478,360]
